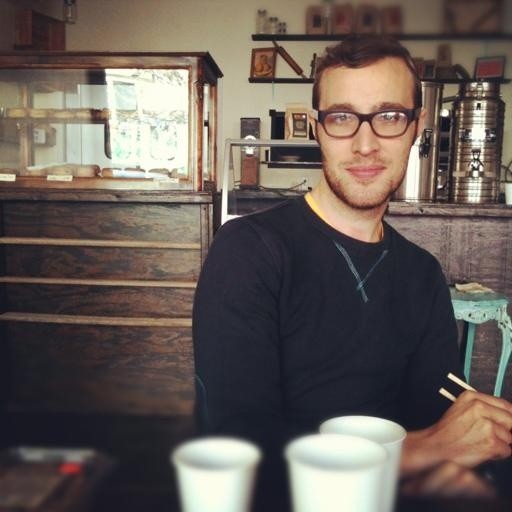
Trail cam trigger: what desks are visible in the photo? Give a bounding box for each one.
[61,439,512,511]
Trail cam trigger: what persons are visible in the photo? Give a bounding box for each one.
[192,35,511,500]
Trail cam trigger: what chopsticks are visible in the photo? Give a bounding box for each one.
[438,373,479,402]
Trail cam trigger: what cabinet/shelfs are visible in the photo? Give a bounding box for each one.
[0,50,224,438]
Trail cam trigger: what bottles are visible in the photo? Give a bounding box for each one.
[322,0,336,36]
[63,1,78,23]
[257,8,288,35]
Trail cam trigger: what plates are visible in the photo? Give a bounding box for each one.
[276,154,302,161]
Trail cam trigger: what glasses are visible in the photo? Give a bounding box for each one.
[307,105,419,140]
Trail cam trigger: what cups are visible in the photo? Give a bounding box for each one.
[170,438,263,512]
[320,412,407,511]
[283,432,389,510]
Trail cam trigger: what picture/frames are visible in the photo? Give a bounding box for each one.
[250,46,277,79]
[473,55,506,80]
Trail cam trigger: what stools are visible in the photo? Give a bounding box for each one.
[448,283,512,397]
